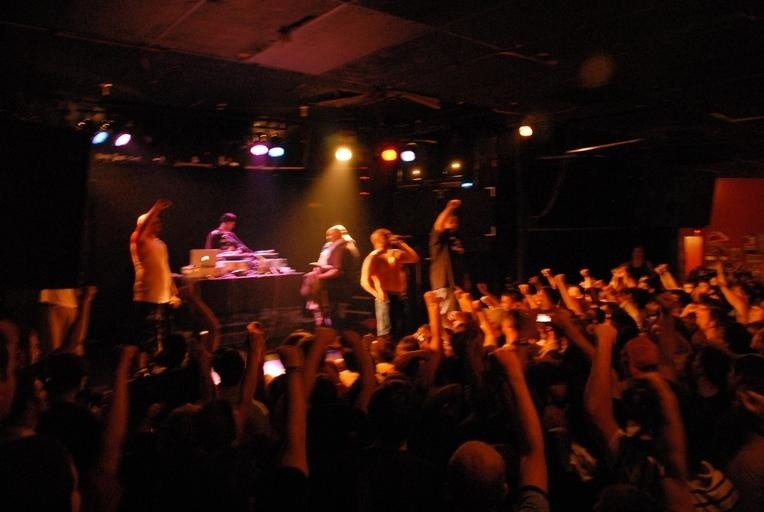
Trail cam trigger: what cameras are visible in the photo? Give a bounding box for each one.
[536,314,552,323]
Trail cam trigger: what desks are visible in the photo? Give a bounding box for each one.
[171,271,307,326]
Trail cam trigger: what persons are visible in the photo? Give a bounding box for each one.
[305,227,336,276]
[359,229,422,334]
[1,236,764,511]
[428,198,464,287]
[206,212,254,253]
[319,225,359,332]
[128,198,179,357]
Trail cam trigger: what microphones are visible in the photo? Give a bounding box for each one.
[388,235,411,241]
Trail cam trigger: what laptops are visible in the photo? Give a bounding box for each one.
[189,249,216,267]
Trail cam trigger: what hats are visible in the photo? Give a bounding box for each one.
[223,214,237,222]
